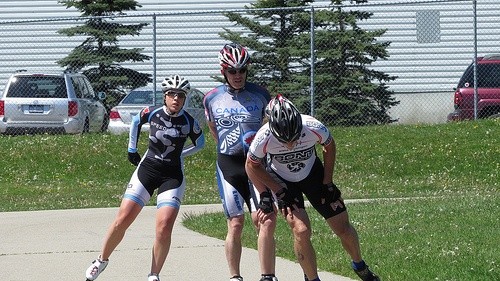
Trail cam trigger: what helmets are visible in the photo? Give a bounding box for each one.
[265,94,302,143]
[218,44,250,69]
[161,75,191,94]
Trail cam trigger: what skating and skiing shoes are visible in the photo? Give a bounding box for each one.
[304,273,321,281]
[148,274,158,281]
[353,260,380,281]
[86,257,107,281]
[229,274,278,281]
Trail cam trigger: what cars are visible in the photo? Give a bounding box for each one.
[109,85,210,135]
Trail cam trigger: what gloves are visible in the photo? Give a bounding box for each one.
[128,152,141,166]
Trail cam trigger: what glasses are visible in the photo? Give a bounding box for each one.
[165,92,186,98]
[224,69,248,74]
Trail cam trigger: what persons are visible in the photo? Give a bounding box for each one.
[245,95,310,281]
[202,44,279,281]
[86,74,205,281]
[246,94,380,281]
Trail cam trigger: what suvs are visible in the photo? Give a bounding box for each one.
[447,54,500,122]
[0,68,109,135]
